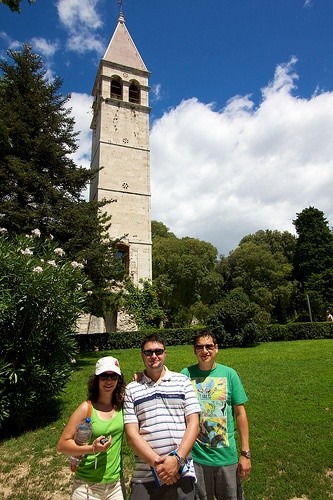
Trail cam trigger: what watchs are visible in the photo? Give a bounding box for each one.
[169,448,186,468]
[241,448,252,458]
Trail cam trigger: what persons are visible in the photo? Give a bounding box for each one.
[57,356,124,500]
[132,329,253,500]
[121,333,201,500]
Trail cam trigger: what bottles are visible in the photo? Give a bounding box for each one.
[70,418,92,463]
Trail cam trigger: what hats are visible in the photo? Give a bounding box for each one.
[95,356,122,375]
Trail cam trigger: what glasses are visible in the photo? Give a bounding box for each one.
[97,372,119,381]
[142,349,165,356]
[194,344,215,350]
